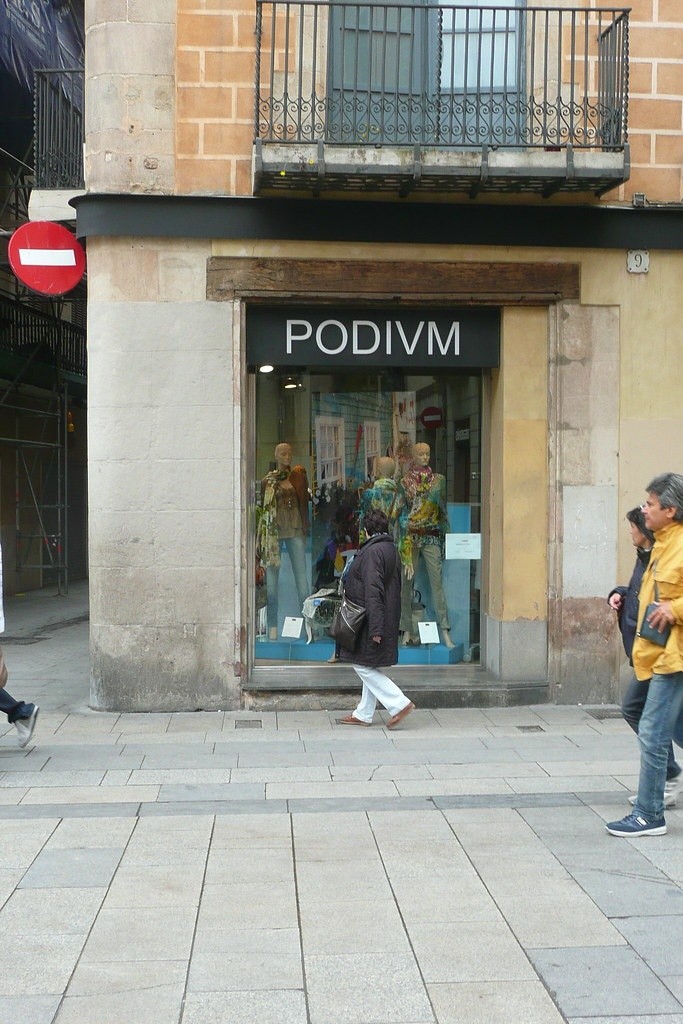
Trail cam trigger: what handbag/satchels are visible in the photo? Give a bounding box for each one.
[328,594,367,651]
[410,591,425,635]
[638,604,671,646]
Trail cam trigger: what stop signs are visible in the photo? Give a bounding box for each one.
[9,221,85,297]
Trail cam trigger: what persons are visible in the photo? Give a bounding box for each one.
[0,542,40,749]
[358,455,412,646]
[605,470,683,839]
[256,442,320,642]
[401,442,457,649]
[335,509,416,728]
[608,505,683,806]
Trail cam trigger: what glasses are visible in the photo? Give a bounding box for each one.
[639,502,672,515]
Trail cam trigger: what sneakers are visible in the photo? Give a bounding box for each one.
[14,706,39,748]
[605,813,668,838]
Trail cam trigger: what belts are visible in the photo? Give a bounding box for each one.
[408,528,439,536]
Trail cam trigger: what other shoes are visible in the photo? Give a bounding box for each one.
[339,714,370,726]
[627,769,683,810]
[386,702,415,728]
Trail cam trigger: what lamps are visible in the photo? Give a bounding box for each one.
[284,375,298,389]
